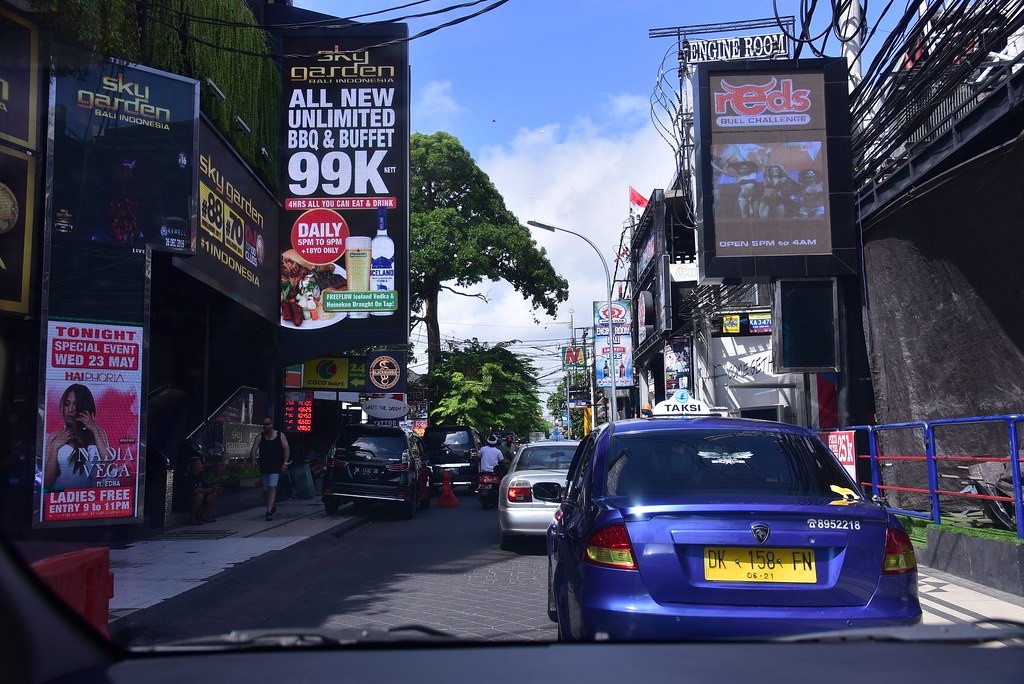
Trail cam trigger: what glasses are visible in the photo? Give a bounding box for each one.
[262,423,272,425]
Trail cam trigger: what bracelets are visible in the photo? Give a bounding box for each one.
[284,463,288,465]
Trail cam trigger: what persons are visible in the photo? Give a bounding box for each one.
[710,161,825,217]
[251,416,289,521]
[521,438,526,444]
[179,457,222,525]
[44,384,108,492]
[496,434,514,470]
[504,435,516,451]
[478,435,508,474]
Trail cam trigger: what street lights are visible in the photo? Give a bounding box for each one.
[531,345,571,441]
[528,219,617,423]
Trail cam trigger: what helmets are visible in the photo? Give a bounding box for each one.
[506,434,514,442]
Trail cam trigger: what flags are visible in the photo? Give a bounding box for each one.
[631,188,648,208]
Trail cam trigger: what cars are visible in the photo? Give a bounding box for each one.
[533,413,921,647]
[422,426,483,493]
[526,434,578,469]
[493,442,581,549]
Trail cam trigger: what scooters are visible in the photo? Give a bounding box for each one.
[472,449,505,509]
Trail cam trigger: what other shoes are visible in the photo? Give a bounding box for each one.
[265,511,273,521]
[188,520,202,525]
[201,517,216,522]
[265,507,277,515]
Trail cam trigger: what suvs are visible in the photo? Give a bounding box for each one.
[322,423,435,518]
